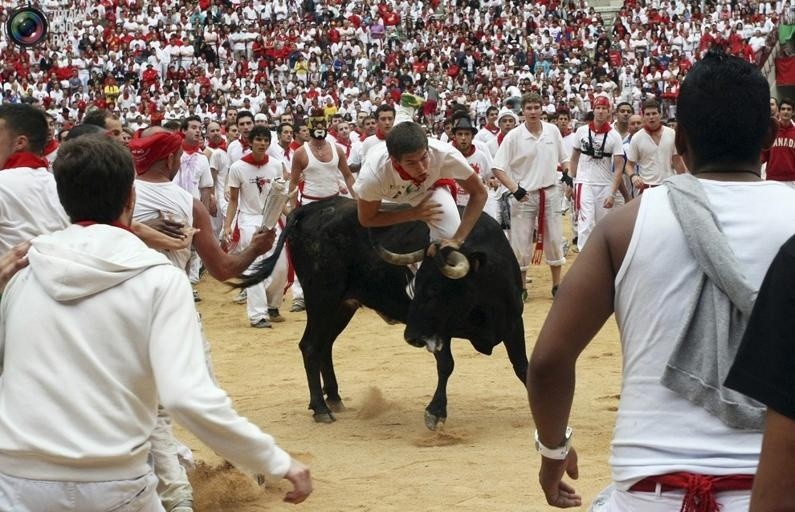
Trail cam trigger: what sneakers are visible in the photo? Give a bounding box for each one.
[233,289,305,328]
[193,289,200,301]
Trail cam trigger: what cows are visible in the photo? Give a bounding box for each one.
[223,195,529,432]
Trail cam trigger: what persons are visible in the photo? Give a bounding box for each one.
[0,0,794,302]
[724,236,795,512]
[147,404,196,512]
[526,49,795,512]
[0,136,314,512]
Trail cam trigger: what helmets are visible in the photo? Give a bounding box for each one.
[592,96,610,110]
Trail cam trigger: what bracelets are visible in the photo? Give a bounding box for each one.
[534,426,572,460]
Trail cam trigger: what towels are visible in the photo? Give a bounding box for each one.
[661,175,768,431]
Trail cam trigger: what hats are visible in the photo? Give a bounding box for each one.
[451,117,477,136]
[493,109,520,128]
